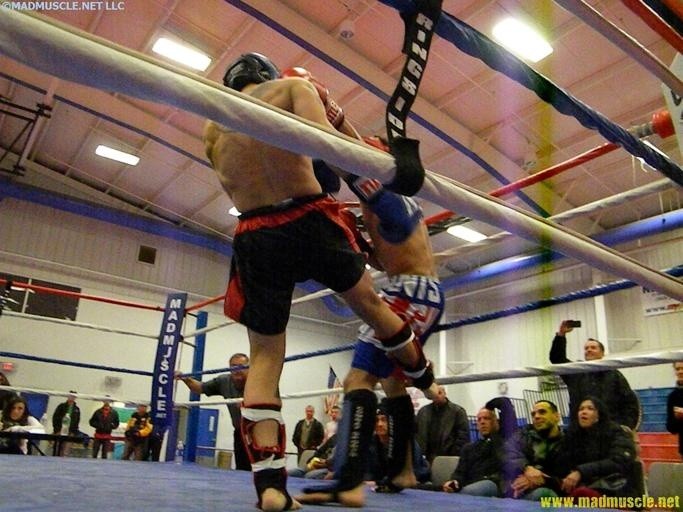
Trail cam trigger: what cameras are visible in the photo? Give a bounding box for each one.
[567,321,581,327]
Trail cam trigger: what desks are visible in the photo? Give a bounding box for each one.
[0,428,89,458]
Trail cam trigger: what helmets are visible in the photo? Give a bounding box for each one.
[223,52,281,92]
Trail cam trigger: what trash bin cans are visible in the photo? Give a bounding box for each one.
[217,451,232,470]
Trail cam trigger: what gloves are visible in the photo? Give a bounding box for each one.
[280,67,344,129]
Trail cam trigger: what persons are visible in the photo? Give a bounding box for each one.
[89,395,120,459]
[665,360,682,461]
[202,51,445,511]
[283,67,445,504]
[173,353,282,471]
[52,390,80,457]
[0,397,45,455]
[121,404,154,461]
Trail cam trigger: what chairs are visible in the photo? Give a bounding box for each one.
[427,455,460,491]
[645,462,681,511]
[296,450,316,470]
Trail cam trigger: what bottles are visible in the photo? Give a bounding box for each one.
[40,413,47,433]
[60,413,71,436]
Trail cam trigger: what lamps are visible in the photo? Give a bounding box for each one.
[521,133,539,168]
[336,0,361,40]
